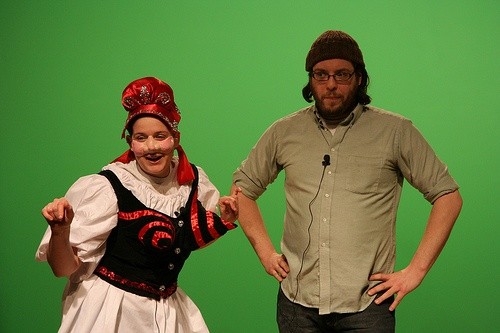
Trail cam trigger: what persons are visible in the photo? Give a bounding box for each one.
[229,30,464,333]
[33,76,242,333]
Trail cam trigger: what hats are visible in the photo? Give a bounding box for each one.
[107,76,195,185]
[306,30,365,71]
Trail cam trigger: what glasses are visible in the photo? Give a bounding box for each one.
[309,68,359,81]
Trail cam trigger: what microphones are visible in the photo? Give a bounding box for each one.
[175,207,185,217]
[322,155,330,166]
